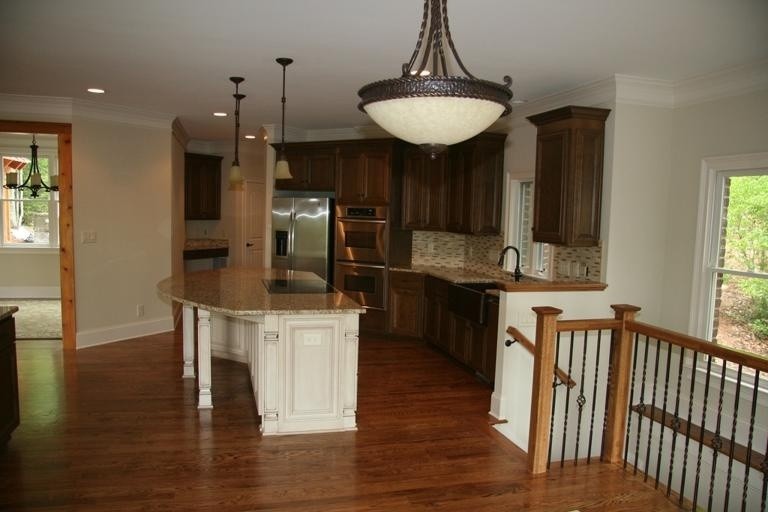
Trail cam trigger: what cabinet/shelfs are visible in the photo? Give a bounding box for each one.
[275,145,335,191]
[402,140,504,235]
[185,158,221,221]
[424,273,499,391]
[337,143,405,205]
[531,118,605,247]
[388,271,424,338]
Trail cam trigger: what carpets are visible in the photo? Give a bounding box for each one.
[0,298,61,341]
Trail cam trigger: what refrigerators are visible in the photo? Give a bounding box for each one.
[271,197,335,282]
[259,278,336,295]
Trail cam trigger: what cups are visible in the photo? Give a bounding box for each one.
[558,257,591,282]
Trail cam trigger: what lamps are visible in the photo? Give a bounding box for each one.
[1,134,59,198]
[357,1,514,161]
[274,58,293,180]
[228,76,247,188]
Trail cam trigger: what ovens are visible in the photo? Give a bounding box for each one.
[336,215,386,309]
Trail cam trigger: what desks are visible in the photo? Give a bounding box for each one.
[0,305,19,442]
